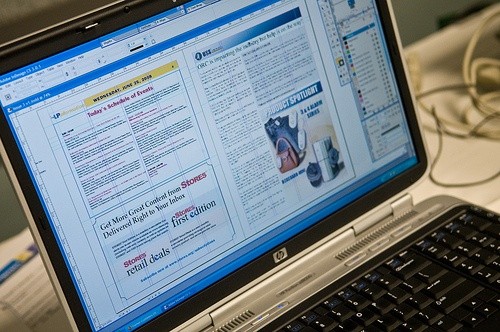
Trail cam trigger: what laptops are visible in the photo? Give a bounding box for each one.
[0,0,500,332]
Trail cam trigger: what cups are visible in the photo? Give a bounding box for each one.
[313,136,339,183]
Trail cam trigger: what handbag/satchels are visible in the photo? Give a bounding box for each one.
[275,137,299,173]
